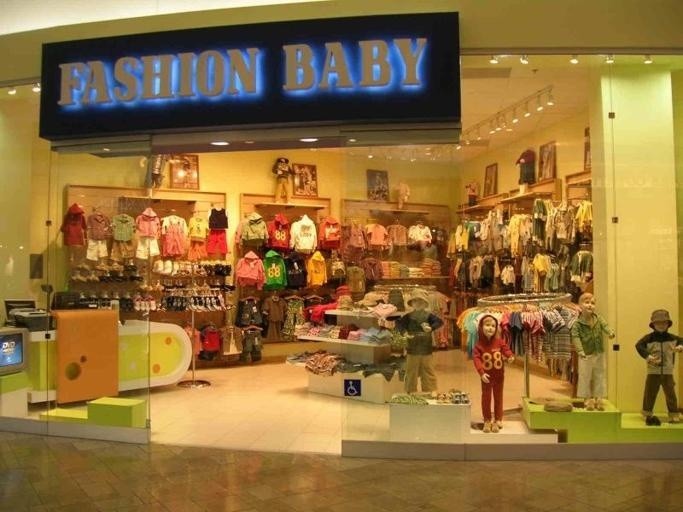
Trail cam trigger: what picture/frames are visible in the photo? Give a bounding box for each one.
[168,155,199,189]
[537,140,556,182]
[481,162,498,197]
[366,169,390,199]
[291,163,318,196]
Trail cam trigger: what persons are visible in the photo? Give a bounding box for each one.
[394,180,410,211]
[142,155,176,198]
[514,149,540,192]
[464,177,480,205]
[397,288,445,394]
[471,315,514,433]
[271,157,297,203]
[635,310,682,423]
[570,293,616,411]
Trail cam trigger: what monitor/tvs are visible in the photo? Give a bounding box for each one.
[0,327,29,376]
[53,292,80,308]
[5,299,35,322]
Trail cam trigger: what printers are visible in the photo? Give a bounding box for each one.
[14,311,56,331]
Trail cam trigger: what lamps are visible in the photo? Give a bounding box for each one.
[433,84,556,160]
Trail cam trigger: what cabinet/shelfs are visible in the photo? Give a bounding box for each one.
[298,305,416,406]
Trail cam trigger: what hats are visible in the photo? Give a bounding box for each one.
[407,288,429,308]
[649,310,672,328]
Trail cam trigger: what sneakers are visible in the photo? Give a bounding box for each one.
[435,388,469,404]
[69,263,142,282]
[118,295,156,312]
[594,401,604,411]
[482,422,491,433]
[494,419,503,429]
[586,399,595,410]
[642,414,652,421]
[668,416,680,423]
